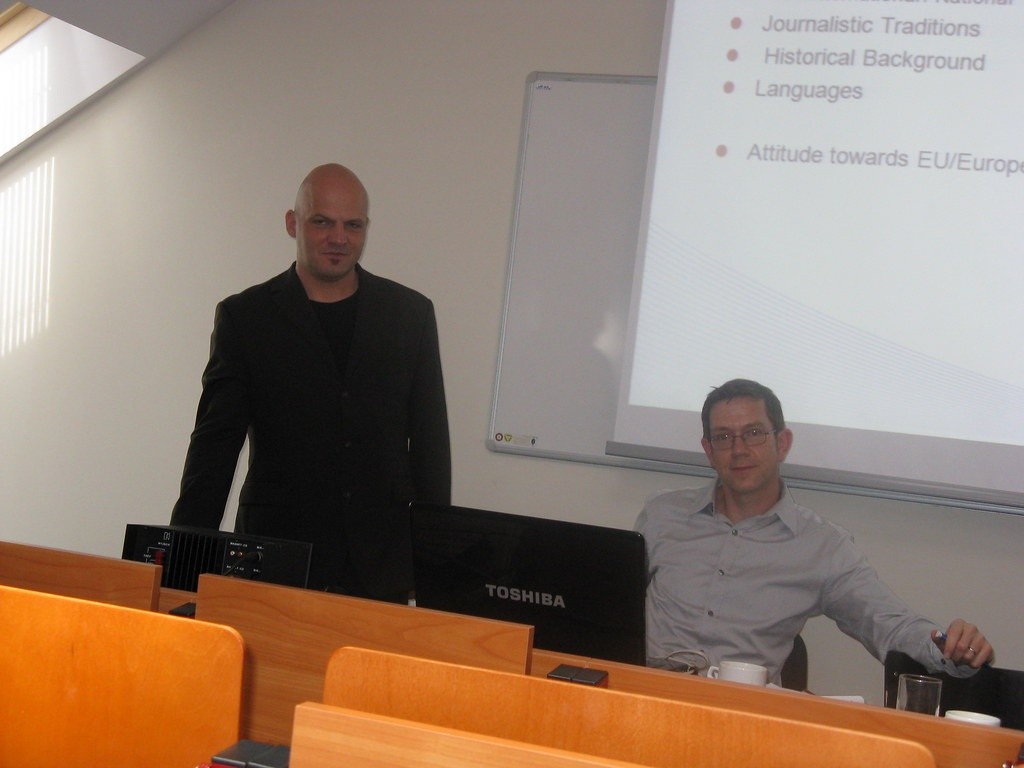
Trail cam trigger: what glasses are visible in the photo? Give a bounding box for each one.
[708,428,778,450]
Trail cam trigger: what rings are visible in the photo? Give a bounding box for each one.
[969,647,977,655]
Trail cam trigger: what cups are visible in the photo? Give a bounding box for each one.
[946,710,1000,727]
[706,661,767,688]
[895,673,942,717]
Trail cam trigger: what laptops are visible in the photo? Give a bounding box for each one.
[121,524,312,594]
[405,503,647,670]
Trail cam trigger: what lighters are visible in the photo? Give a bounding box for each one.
[935,631,946,641]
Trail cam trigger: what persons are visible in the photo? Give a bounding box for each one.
[169,163,451,607]
[633,379,993,690]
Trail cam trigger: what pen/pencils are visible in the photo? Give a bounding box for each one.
[935,630,994,673]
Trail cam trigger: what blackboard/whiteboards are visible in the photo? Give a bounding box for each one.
[484,71,1024,516]
